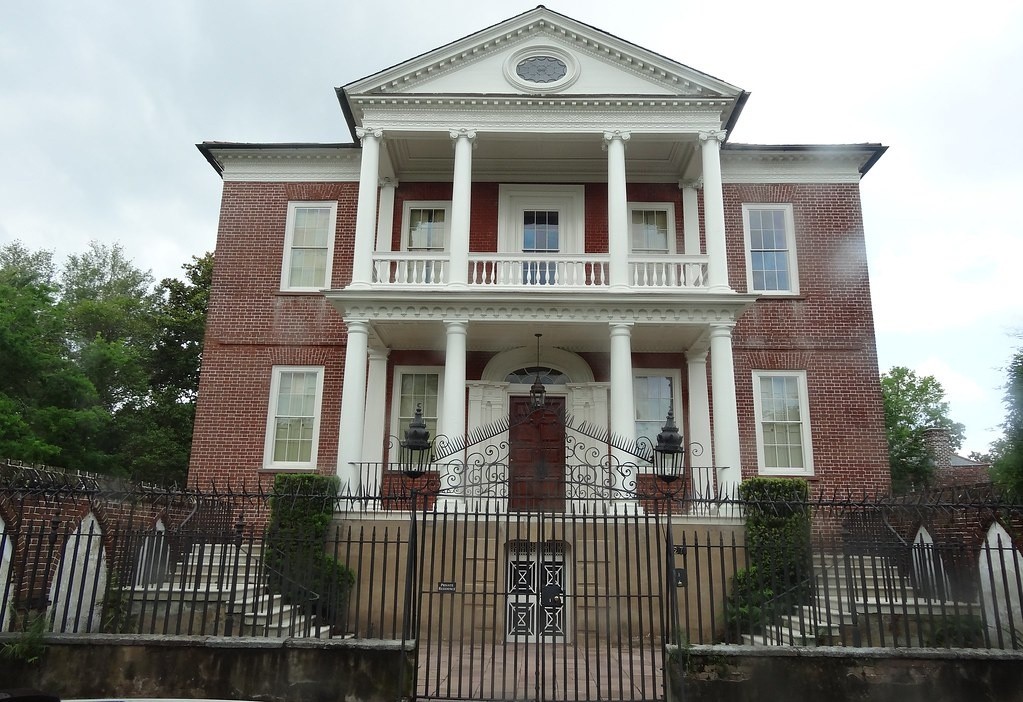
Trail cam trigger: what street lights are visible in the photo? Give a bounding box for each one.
[397,401,432,641]
[652,405,685,647]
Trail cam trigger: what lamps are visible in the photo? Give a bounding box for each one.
[531,333,546,407]
